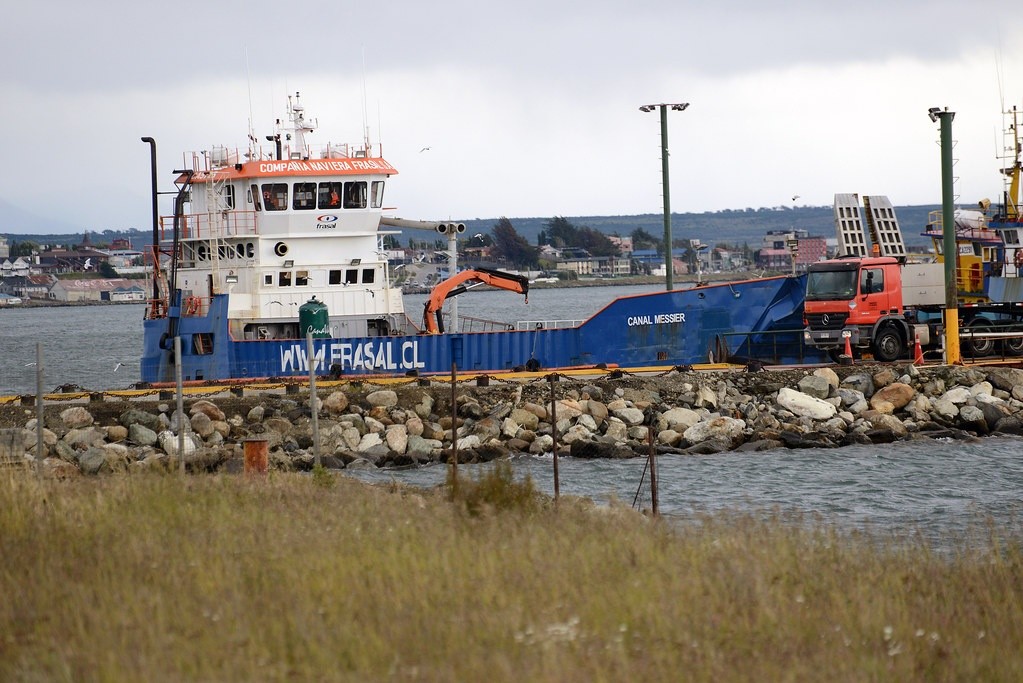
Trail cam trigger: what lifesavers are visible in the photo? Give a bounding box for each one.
[186,296,198,315]
[525,359,539,371]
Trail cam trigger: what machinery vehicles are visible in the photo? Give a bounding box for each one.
[415,265,530,336]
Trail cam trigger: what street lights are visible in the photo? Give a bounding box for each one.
[690,238,709,287]
[638,103,690,291]
[785,232,799,273]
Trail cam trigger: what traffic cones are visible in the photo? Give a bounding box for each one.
[913,333,926,367]
[844,335,854,365]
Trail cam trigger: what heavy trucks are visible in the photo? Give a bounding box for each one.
[800,255,1023,364]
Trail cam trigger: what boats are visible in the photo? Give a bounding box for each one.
[919,55,1023,301]
[140,48,408,384]
